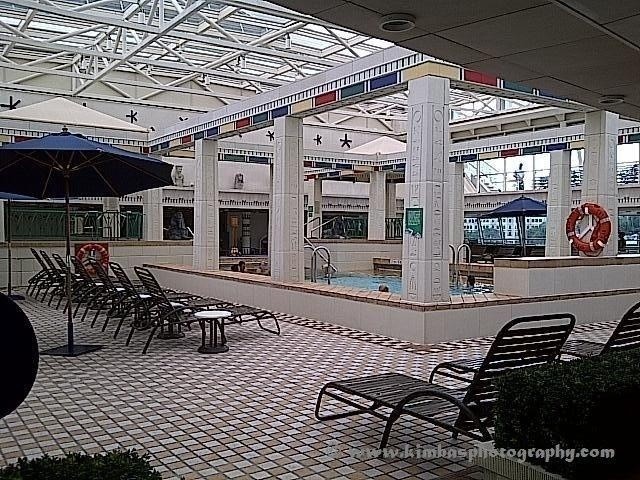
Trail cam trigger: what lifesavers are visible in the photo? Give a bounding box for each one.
[76,244,107,276]
[566,203,611,252]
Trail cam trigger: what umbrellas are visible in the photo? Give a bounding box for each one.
[482,195,547,257]
[0,124,174,322]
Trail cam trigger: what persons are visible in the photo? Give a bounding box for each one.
[173,166,185,186]
[464,275,475,289]
[513,163,524,190]
[231,261,245,272]
[379,285,389,292]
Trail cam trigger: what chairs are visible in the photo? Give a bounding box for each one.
[314,295,639,460]
[24,244,282,355]
[471,244,534,264]
